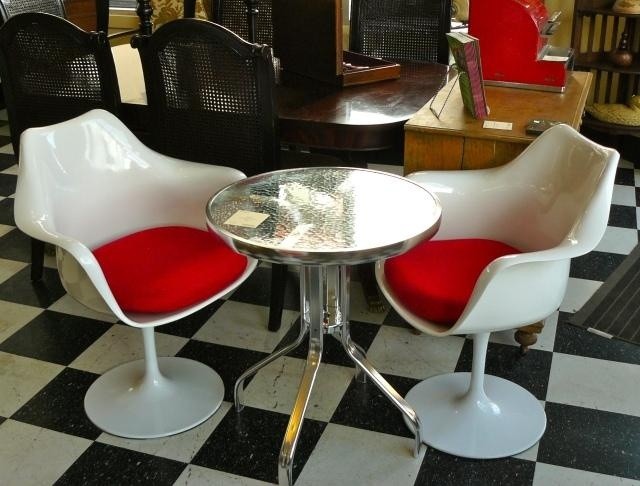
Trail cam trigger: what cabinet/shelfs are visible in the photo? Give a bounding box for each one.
[569,1,639,168]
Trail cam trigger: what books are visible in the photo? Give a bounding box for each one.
[446,31,488,120]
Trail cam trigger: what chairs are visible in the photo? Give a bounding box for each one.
[210,0,280,61]
[0,0,68,20]
[348,0,451,66]
[0,9,157,282]
[11,106,259,441]
[376,122,622,461]
[129,16,346,332]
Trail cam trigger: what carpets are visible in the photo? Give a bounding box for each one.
[566,244,640,347]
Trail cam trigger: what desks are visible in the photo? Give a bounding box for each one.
[23,42,460,171]
[205,165,443,486]
[403,71,595,178]
